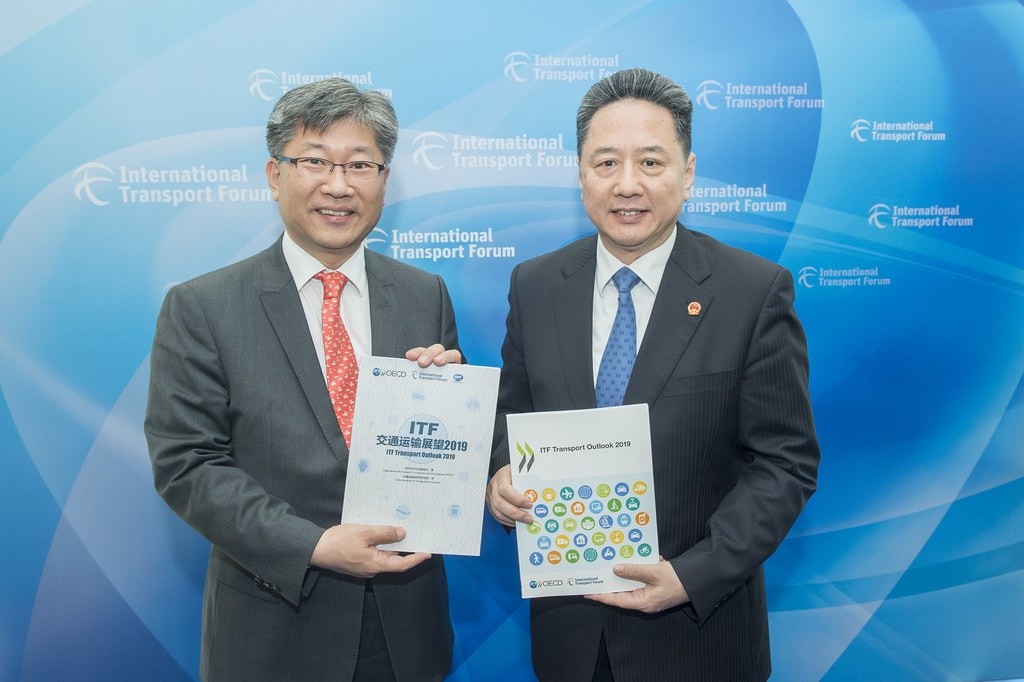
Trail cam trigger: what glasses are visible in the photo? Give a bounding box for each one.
[275,154,389,182]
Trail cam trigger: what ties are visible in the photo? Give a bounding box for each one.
[312,270,359,451]
[595,268,642,408]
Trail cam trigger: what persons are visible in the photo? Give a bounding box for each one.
[144,78,468,682]
[486,68,820,682]
